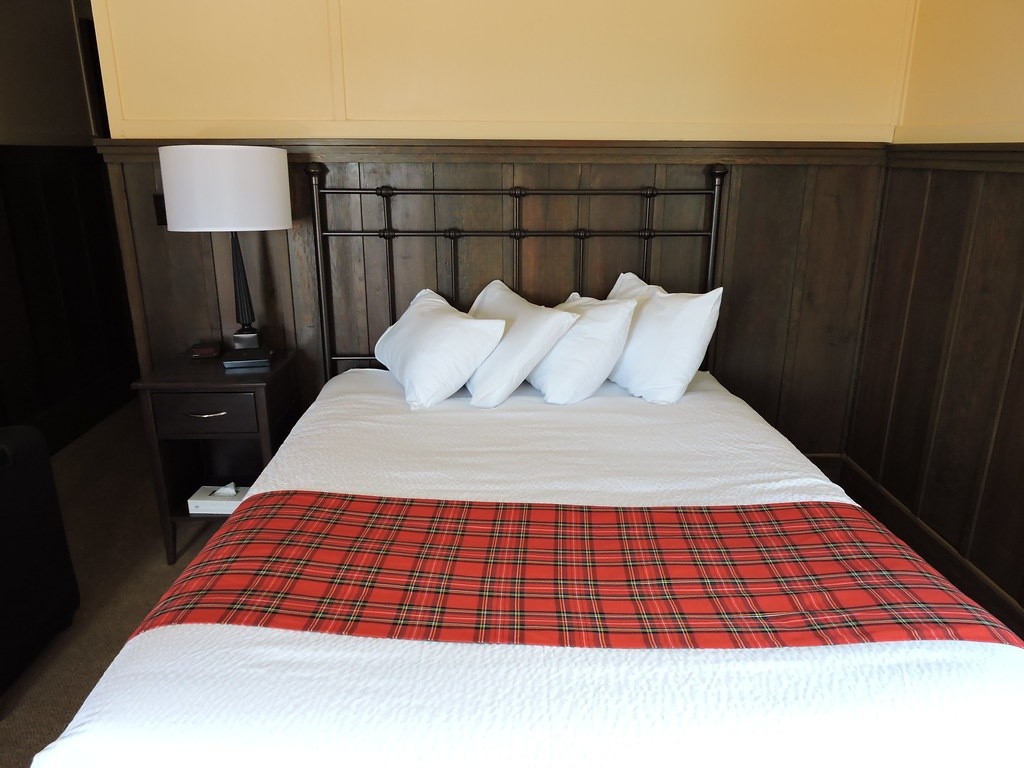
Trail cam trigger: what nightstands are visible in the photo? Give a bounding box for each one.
[130,349,297,566]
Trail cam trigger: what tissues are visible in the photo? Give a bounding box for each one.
[187,481,253,515]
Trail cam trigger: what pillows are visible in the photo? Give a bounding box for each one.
[607,272,724,405]
[523,293,637,405]
[463,280,580,409]
[374,288,506,410]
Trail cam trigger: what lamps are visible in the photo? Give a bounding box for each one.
[157,143,293,370]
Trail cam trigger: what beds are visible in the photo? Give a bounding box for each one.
[29,161,1024,768]
